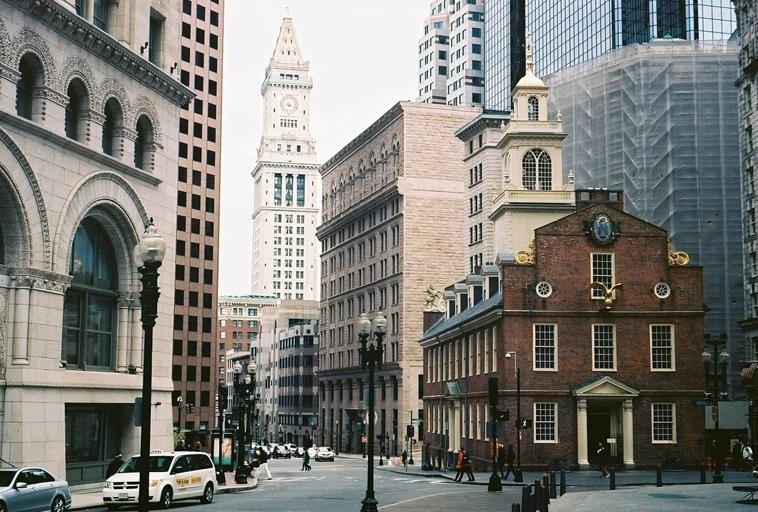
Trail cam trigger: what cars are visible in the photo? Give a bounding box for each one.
[249,440,336,462]
[0,466,73,512]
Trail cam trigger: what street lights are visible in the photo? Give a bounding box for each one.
[310,421,314,447]
[361,419,367,459]
[352,306,390,512]
[130,214,169,512]
[175,395,183,433]
[700,348,730,484]
[231,357,262,484]
[335,420,339,455]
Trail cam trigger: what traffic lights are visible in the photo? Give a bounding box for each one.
[407,426,414,437]
[411,439,416,444]
[494,409,510,422]
[186,403,190,414]
[519,418,533,428]
[189,404,194,414]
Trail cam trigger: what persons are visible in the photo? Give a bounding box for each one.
[455,446,475,482]
[595,441,611,479]
[255,447,273,481]
[503,443,516,481]
[451,449,472,481]
[708,437,756,475]
[496,443,507,479]
[174,439,202,452]
[300,447,312,471]
[402,449,407,466]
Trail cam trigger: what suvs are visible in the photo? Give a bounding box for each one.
[101,448,219,512]
